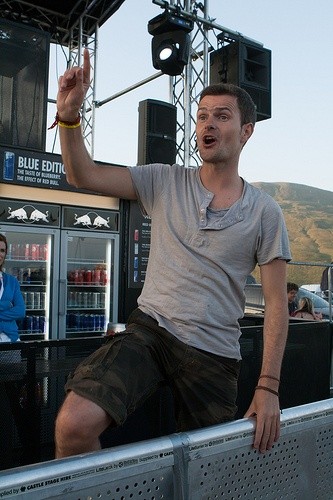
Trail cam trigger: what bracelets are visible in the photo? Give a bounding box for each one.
[258,375,280,383]
[48,111,81,130]
[254,386,279,397]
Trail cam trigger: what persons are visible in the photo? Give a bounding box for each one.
[287,283,298,316]
[0,234,25,365]
[54,49,291,462]
[291,297,321,320]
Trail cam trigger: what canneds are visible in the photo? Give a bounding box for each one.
[1,267,47,333]
[5,243,48,261]
[64,269,107,331]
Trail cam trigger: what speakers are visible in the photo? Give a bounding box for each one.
[210,40,271,122]
[137,99,177,166]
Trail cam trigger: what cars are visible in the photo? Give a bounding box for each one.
[242,284,332,327]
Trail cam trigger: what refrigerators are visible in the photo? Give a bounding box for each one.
[0,144,126,345]
